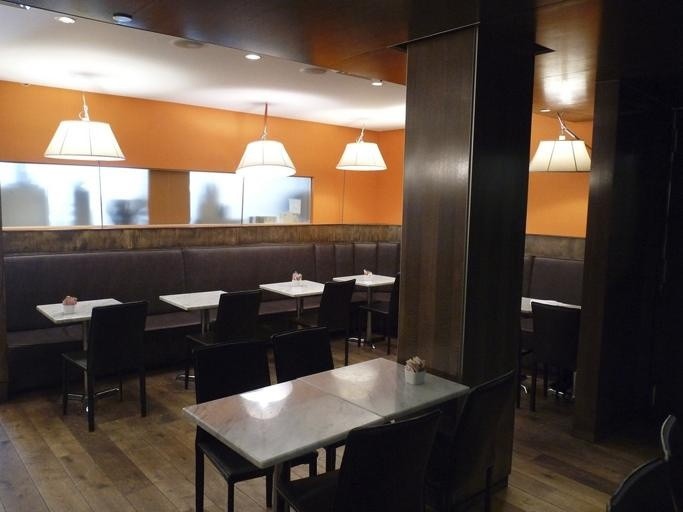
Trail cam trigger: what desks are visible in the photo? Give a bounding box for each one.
[159,290,227,382]
[36,298,124,417]
[521,297,586,395]
[333,275,397,352]
[259,280,325,331]
[182,358,470,511]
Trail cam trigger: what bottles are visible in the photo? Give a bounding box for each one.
[292,273,303,286]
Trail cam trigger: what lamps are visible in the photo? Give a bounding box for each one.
[335,123,388,173]
[528,110,592,173]
[42,94,127,165]
[233,102,296,182]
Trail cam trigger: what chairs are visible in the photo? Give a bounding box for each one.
[184,289,334,512]
[607,415,683,512]
[358,274,401,355]
[61,301,151,432]
[276,370,520,510]
[531,302,584,411]
[296,279,357,366]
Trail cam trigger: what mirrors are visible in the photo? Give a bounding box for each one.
[188,171,311,224]
[1,160,150,226]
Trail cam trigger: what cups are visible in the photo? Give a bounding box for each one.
[404,369,424,385]
[61,303,74,314]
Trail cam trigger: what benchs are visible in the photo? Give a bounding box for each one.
[4,243,402,402]
[520,255,586,352]
